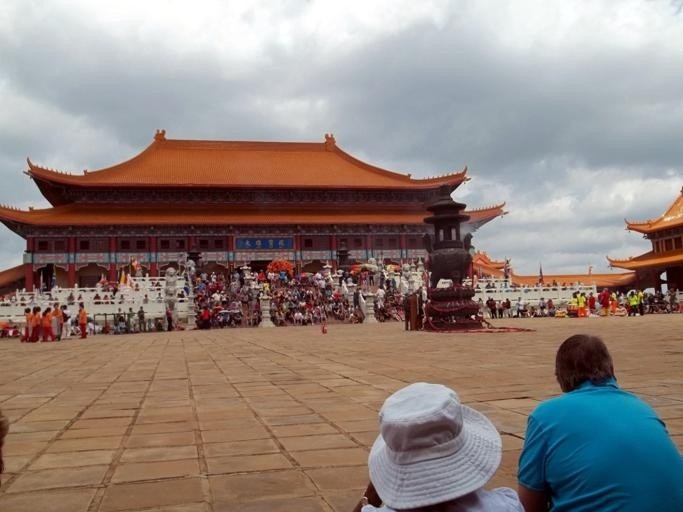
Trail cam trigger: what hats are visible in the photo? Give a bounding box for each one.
[368,382,502,510]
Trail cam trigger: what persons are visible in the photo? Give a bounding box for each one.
[515,335,683,511]
[473,280,676,318]
[194,264,428,329]
[178,291,185,303]
[354,381,525,511]
[21,280,173,341]
